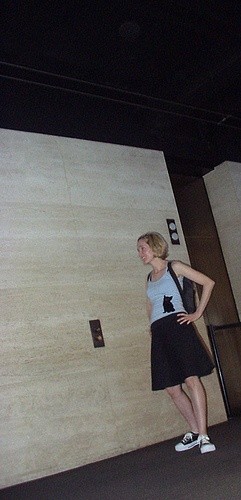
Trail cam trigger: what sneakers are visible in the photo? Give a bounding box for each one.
[199,434,216,454]
[175,431,199,451]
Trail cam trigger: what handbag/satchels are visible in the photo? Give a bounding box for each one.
[147,259,197,314]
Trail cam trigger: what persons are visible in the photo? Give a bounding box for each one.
[137,231,217,455]
